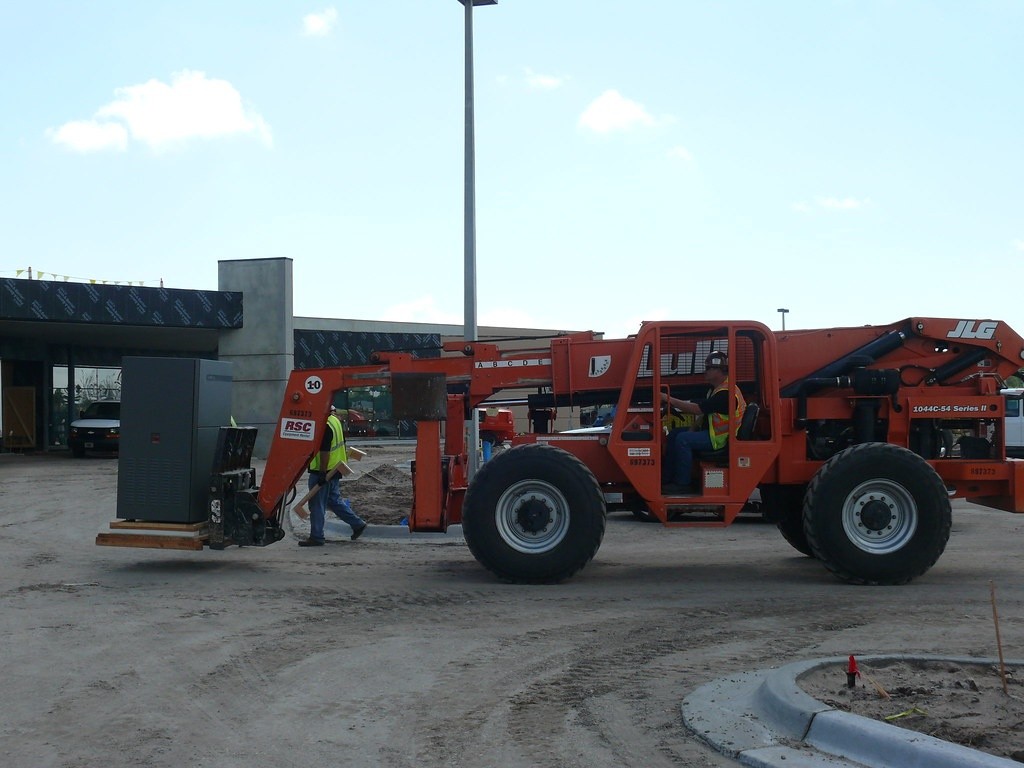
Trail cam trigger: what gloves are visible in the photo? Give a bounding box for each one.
[309,471,326,491]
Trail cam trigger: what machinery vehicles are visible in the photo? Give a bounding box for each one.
[204,316,1024,588]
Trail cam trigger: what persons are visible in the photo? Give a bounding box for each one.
[660,350,747,495]
[297,404,368,547]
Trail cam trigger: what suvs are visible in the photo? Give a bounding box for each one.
[68,400,120,456]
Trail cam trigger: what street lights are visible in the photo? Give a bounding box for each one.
[457,0,499,490]
[777,309,789,330]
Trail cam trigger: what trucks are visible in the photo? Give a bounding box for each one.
[969,388,1024,454]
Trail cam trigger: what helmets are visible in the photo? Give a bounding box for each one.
[705,351,728,367]
[330,405,336,412]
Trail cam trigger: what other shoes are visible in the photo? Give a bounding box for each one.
[351,523,366,540]
[298,537,323,546]
[662,484,689,494]
